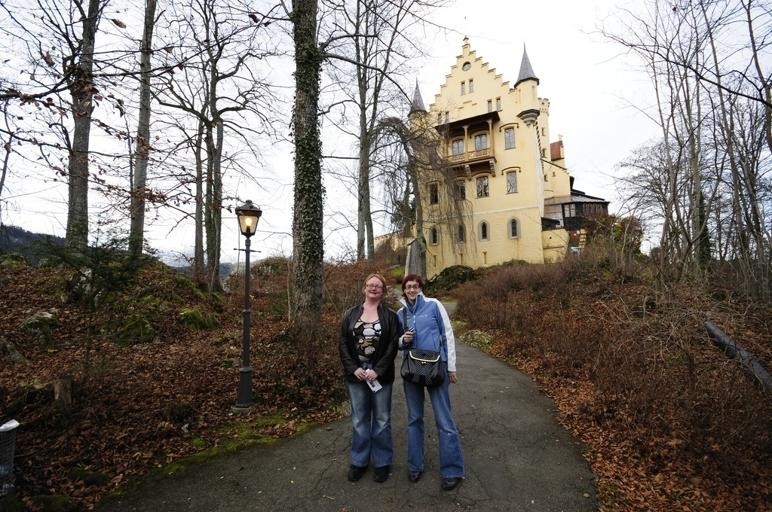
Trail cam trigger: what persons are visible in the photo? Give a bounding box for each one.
[396,273,465,490]
[337,273,400,483]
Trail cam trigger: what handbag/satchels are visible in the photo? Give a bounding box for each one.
[401,349,445,387]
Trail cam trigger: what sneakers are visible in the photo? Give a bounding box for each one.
[348,465,368,482]
[373,466,389,483]
[441,478,460,491]
[409,471,421,483]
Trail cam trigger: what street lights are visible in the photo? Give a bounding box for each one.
[230,200,263,413]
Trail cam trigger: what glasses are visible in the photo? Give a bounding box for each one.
[365,284,383,289]
